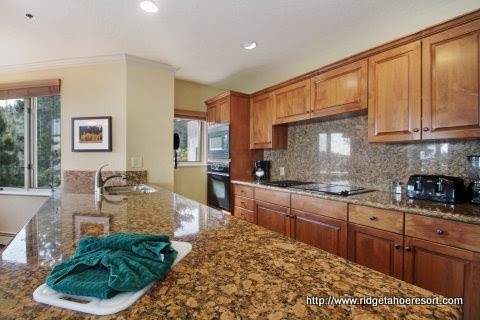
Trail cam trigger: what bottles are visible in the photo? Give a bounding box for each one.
[395,180,402,194]
[390,181,396,194]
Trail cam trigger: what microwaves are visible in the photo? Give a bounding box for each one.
[207,130,229,155]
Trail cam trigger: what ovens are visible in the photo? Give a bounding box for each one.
[206,162,231,211]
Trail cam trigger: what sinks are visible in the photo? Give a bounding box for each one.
[103,183,159,194]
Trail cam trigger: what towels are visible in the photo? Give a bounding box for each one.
[43,234,179,300]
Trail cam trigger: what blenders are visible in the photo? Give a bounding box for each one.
[465,155,480,204]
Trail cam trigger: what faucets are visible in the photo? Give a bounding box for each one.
[93,163,129,196]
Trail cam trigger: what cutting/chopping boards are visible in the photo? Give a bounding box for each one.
[33,240,193,316]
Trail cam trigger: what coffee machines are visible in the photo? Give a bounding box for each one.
[252,159,270,183]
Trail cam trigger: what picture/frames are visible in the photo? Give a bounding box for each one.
[70,116,112,152]
[72,214,113,246]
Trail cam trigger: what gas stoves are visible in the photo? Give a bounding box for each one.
[264,179,313,187]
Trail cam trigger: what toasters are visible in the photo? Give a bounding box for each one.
[405,174,466,204]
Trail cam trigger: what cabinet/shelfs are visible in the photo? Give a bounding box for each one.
[206,97,231,124]
[367,21,480,141]
[248,92,275,149]
[348,202,480,319]
[273,58,369,127]
[255,187,346,261]
[231,184,253,223]
